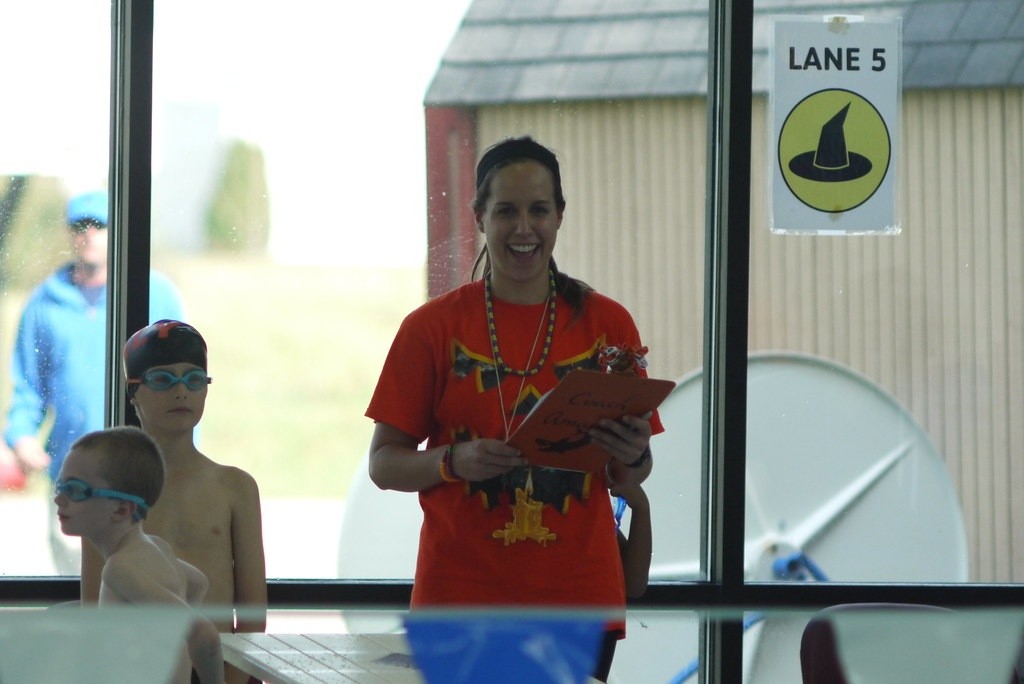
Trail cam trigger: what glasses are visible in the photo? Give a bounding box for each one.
[71,220,105,232]
[127,370,213,391]
[55,479,148,510]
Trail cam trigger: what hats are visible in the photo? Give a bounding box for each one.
[123,319,207,399]
[68,192,108,224]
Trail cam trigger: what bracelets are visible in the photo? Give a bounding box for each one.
[605,464,614,486]
[624,446,651,468]
[439,439,462,483]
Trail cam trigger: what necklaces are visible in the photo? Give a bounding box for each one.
[483,268,557,376]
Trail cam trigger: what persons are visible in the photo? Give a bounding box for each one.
[3,189,267,684]
[364,136,666,684]
